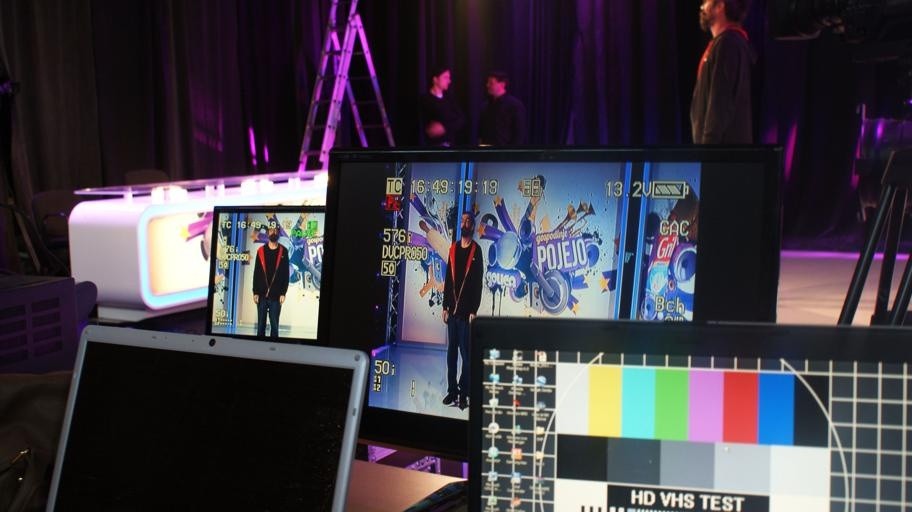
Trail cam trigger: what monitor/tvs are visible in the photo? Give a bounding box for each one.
[45,325,369,511]
[465,314,911,511]
[318,144,779,463]
[203,204,324,342]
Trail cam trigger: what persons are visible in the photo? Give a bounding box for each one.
[685,0,756,147]
[251,217,290,338]
[441,211,484,409]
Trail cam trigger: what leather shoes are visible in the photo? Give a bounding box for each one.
[457,394,468,410]
[443,391,458,405]
